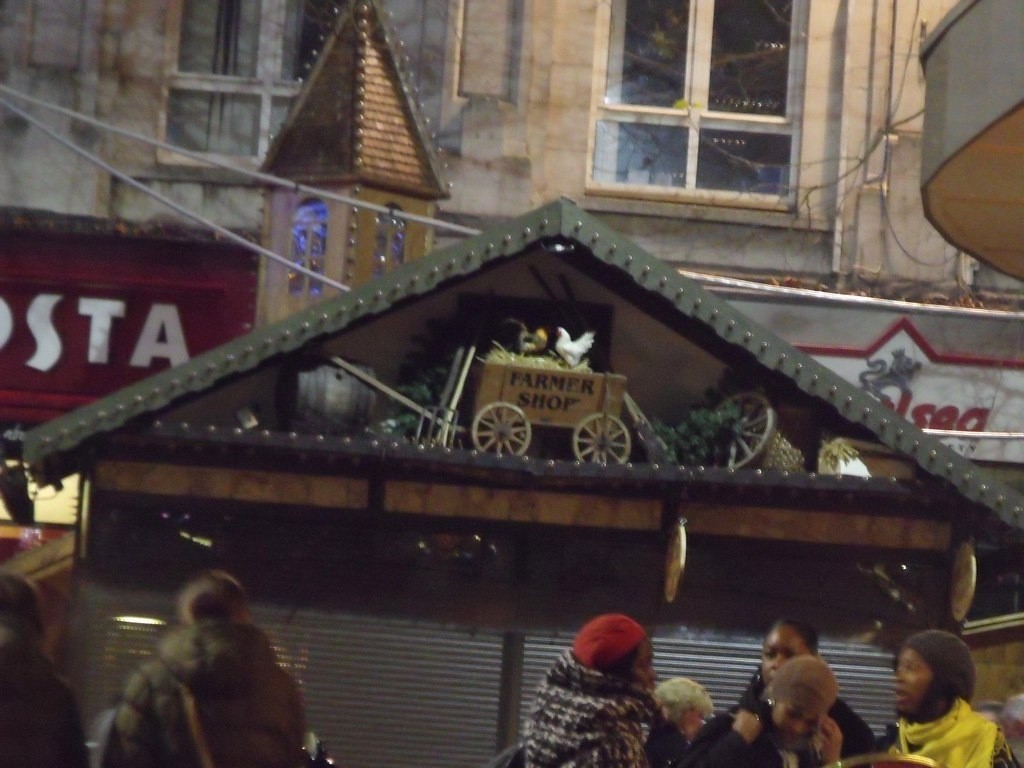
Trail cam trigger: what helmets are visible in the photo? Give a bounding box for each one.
[656,677,714,714]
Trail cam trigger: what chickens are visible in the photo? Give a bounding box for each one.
[502,316,549,357]
[554,326,596,370]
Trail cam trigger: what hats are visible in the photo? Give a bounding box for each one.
[887,629,976,721]
[575,614,646,669]
[773,657,841,717]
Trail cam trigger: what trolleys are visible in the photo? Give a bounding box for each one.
[329,341,633,466]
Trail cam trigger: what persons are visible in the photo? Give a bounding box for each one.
[0,571,94,768]
[108,566,309,768]
[875,628,1022,768]
[524,616,666,768]
[644,613,875,768]
